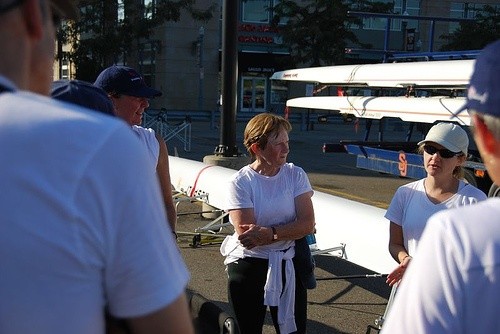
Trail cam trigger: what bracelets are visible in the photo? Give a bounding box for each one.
[270,226,278,243]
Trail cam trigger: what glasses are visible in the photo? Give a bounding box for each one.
[423,145,461,158]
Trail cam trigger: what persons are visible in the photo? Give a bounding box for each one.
[375,36,500,334]
[386,120,491,283]
[51,78,117,117]
[0,0,193,334]
[91,63,177,240]
[225,112,314,334]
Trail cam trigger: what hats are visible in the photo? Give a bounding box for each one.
[417,122,469,153]
[93,65,164,98]
[52,79,114,116]
[448,39,499,119]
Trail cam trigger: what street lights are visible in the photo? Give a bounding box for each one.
[198,26,205,111]
[401,12,408,63]
